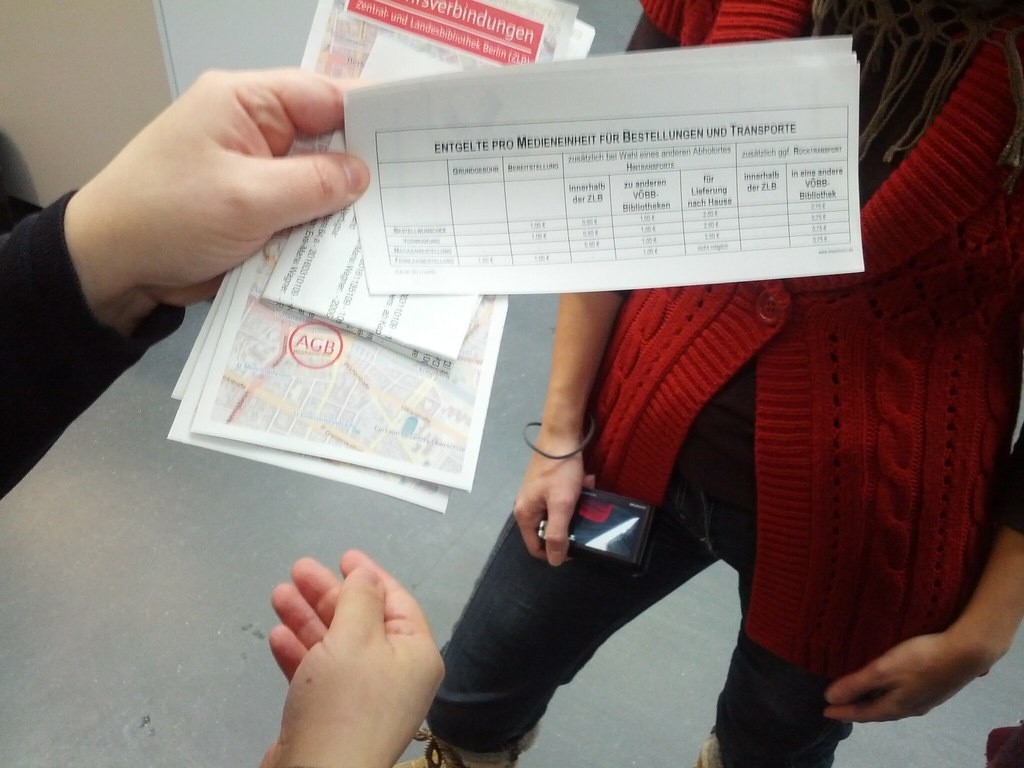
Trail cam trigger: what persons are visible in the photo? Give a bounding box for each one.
[0,62,445,768]
[392,2,1024,768]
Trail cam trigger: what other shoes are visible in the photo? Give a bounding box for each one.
[393,719,542,768]
[695,733,725,768]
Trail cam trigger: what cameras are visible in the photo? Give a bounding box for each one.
[538,486,653,567]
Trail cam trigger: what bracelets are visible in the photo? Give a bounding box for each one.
[522,418,596,461]
